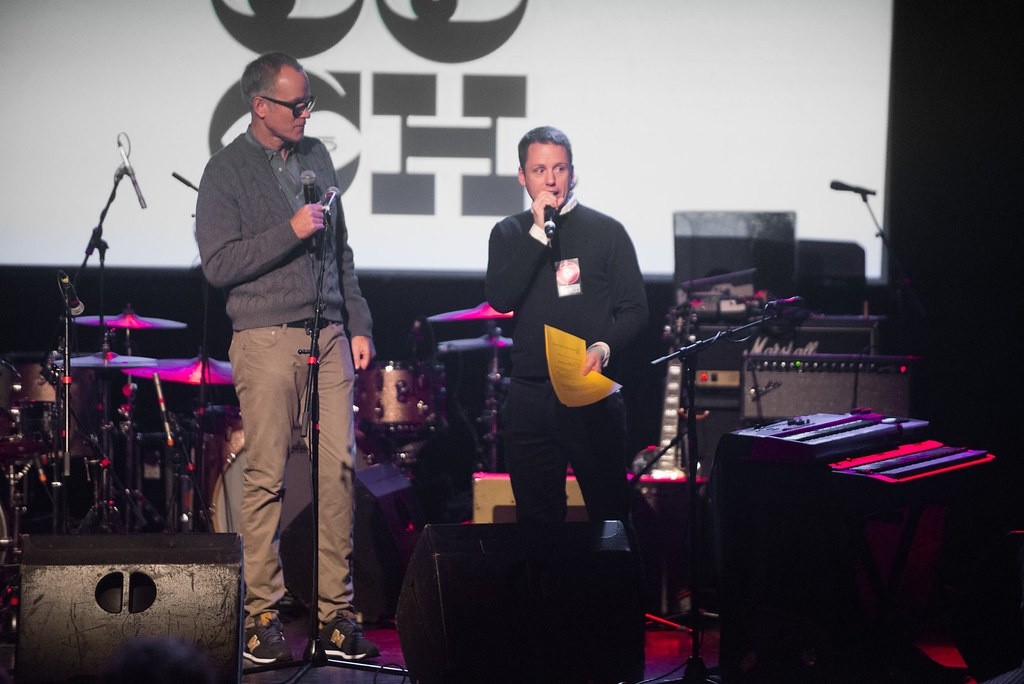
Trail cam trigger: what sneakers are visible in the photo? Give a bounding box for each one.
[243,611,293,664]
[311,615,382,662]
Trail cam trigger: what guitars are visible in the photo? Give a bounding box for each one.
[632,300,704,505]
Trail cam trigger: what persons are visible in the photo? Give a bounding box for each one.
[193,54,382,662]
[484,126,650,533]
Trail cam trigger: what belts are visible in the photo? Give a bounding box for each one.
[273,317,337,330]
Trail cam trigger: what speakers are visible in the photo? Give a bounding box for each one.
[670,233,868,484]
[393,521,645,684]
[19,532,247,684]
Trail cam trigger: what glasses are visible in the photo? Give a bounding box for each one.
[260,95,316,118]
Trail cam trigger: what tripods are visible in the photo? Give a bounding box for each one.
[641,309,783,684]
[241,213,415,684]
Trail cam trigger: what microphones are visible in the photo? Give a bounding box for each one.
[117,141,147,209]
[830,180,877,196]
[544,204,557,238]
[301,168,319,204]
[322,185,342,214]
[59,269,84,316]
[767,295,807,310]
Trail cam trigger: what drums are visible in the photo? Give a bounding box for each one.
[200,411,368,539]
[359,358,431,436]
[4,350,98,426]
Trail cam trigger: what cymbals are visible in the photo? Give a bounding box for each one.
[52,351,158,369]
[119,356,238,386]
[438,334,514,351]
[71,313,190,329]
[428,302,516,322]
